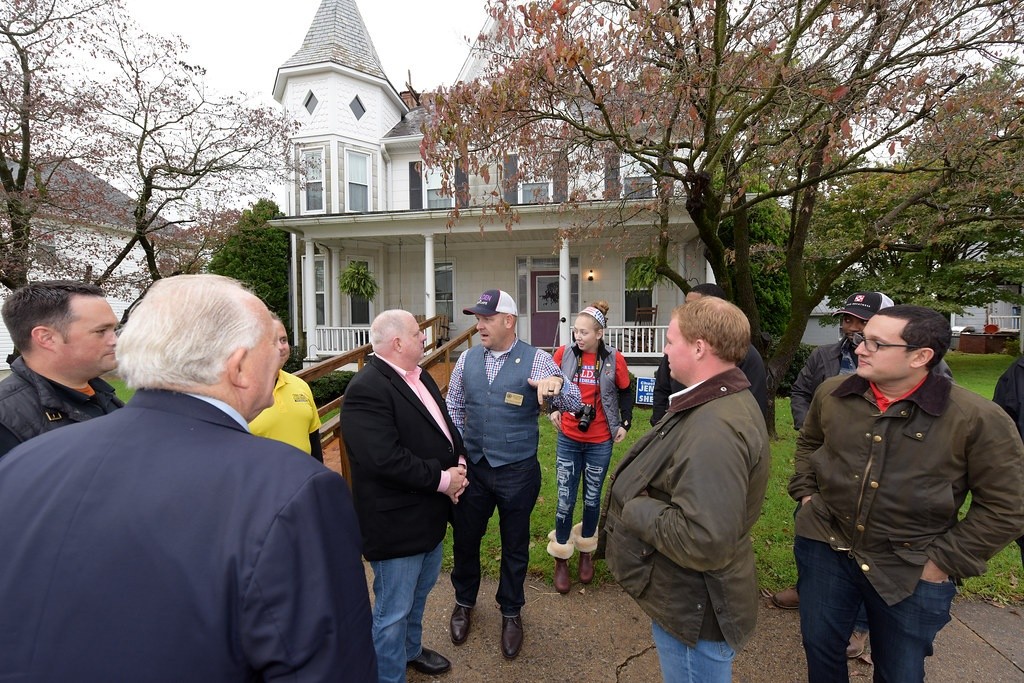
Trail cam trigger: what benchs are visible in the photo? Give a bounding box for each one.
[413,315,450,349]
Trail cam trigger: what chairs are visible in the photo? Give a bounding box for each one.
[621,305,659,353]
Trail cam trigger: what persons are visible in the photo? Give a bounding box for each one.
[0,279,121,459]
[340,309,469,683]
[787,305,1024,683]
[651,282,768,442]
[548,301,636,594]
[247,312,323,465]
[992,355,1024,573]
[446,289,583,660]
[774,291,953,658]
[592,296,771,683]
[0,274,380,683]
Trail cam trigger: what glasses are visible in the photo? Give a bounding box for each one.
[852,333,920,353]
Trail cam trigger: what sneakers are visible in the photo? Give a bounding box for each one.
[846,629,870,657]
[773,585,801,608]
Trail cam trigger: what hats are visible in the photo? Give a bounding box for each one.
[462,289,518,318]
[832,292,895,323]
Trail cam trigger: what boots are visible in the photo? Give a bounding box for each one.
[547,527,573,594]
[572,521,600,583]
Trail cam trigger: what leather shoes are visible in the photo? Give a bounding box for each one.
[405,648,452,675]
[501,613,523,659]
[450,603,470,644]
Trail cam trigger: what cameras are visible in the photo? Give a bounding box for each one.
[575,404,596,432]
